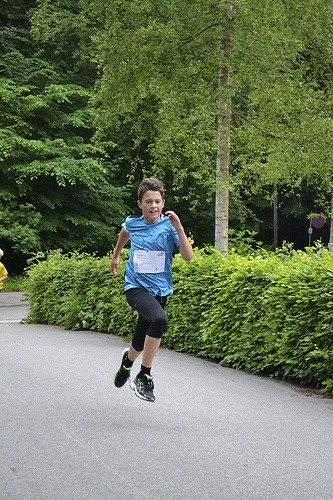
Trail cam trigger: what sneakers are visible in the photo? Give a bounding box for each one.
[130,373,155,402]
[114,348,133,388]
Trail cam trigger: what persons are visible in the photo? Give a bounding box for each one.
[0,248,7,293]
[111,178,192,403]
